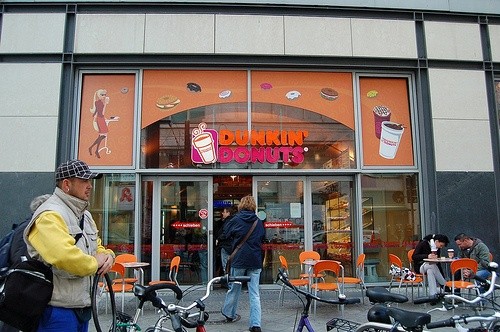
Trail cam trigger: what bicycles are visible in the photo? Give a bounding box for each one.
[276,265,500,332]
[132,269,250,332]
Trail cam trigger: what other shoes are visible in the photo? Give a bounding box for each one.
[440,285,450,292]
[249,326,261,332]
[472,304,486,310]
[430,301,436,306]
[447,299,461,304]
[221,308,233,321]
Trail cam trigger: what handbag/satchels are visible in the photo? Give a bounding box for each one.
[225,256,232,274]
[0,260,53,332]
[389,264,415,281]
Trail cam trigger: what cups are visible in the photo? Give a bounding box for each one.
[447,249,454,259]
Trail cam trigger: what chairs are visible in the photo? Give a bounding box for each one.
[276,251,366,315]
[94,253,181,317]
[388,250,492,315]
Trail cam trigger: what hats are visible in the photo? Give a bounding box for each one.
[55,160,103,180]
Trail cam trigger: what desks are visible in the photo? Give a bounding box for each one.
[422,258,466,307]
[299,259,341,316]
[114,261,149,315]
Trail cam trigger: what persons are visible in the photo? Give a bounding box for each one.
[10,194,53,265]
[454,233,490,294]
[412,234,450,305]
[23,160,115,332]
[218,196,264,332]
[198,205,238,285]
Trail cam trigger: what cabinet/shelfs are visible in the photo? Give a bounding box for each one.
[325,196,373,263]
